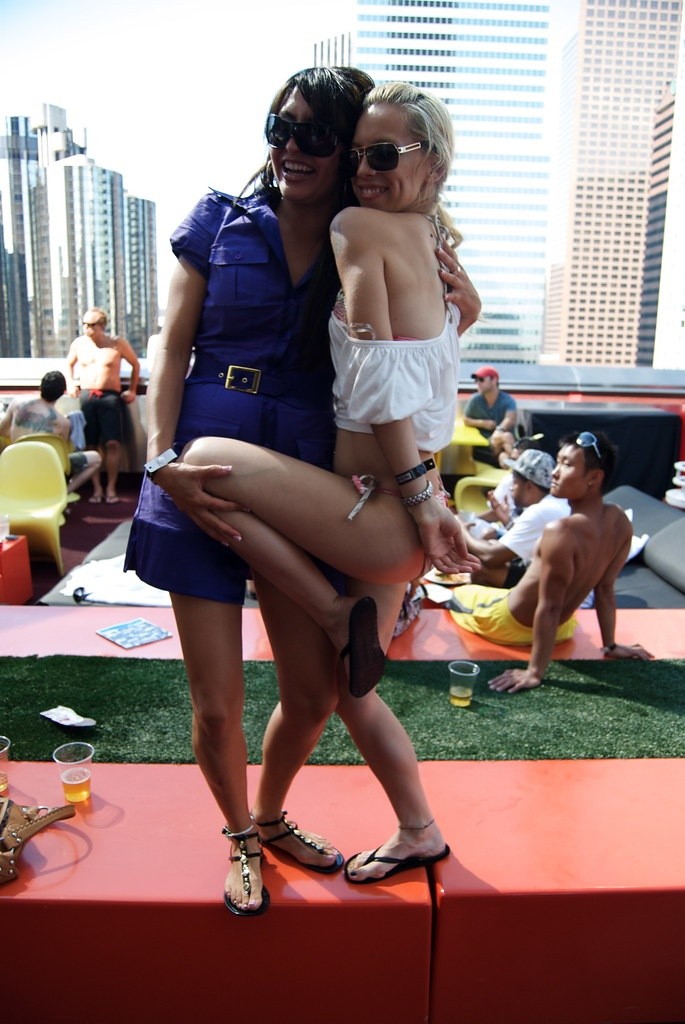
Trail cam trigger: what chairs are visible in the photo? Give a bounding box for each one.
[0,431,69,581]
[454,466,513,513]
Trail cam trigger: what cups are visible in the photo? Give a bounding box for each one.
[0,734,11,793]
[53,742,94,801]
[447,660,480,707]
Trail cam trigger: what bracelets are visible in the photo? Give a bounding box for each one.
[496,425,505,433]
[401,480,433,507]
[396,456,434,485]
[505,520,515,532]
[601,644,616,653]
[144,448,177,482]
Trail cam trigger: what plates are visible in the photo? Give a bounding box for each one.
[422,567,471,585]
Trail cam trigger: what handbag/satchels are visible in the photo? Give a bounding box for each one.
[0,797,76,884]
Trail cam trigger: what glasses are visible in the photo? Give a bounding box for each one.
[264,112,341,158]
[576,431,601,460]
[82,321,99,328]
[475,378,484,383]
[345,140,426,176]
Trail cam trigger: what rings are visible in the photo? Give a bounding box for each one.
[452,267,462,276]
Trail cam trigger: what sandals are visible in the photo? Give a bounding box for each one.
[222,814,270,916]
[250,810,346,875]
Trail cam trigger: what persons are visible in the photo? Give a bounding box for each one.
[0,371,103,512]
[465,367,519,470]
[448,427,655,695]
[63,308,140,504]
[452,436,572,589]
[174,85,481,885]
[122,70,481,917]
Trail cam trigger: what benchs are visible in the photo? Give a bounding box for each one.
[0,758,685,1024]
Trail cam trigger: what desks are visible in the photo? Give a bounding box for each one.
[0,535,33,605]
[425,413,487,476]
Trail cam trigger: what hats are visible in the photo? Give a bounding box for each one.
[503,448,556,489]
[471,365,499,382]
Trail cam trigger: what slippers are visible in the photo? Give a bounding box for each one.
[344,842,450,884]
[105,495,119,504]
[88,494,104,503]
[339,596,384,697]
